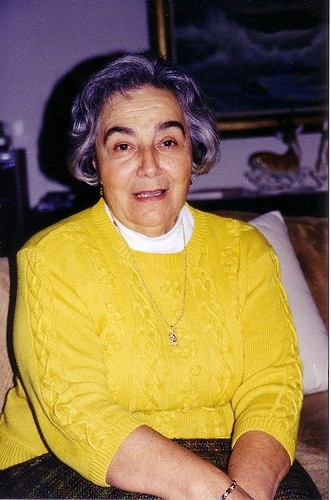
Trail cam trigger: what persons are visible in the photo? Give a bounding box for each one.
[0,52,322,499]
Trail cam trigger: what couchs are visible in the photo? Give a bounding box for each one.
[211,211,329,500]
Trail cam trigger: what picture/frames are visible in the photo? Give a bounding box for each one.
[145,0,329,140]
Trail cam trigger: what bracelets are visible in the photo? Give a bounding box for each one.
[219,480,238,499]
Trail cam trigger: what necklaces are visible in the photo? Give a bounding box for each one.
[107,210,187,349]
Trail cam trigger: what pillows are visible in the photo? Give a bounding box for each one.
[247,212,328,395]
